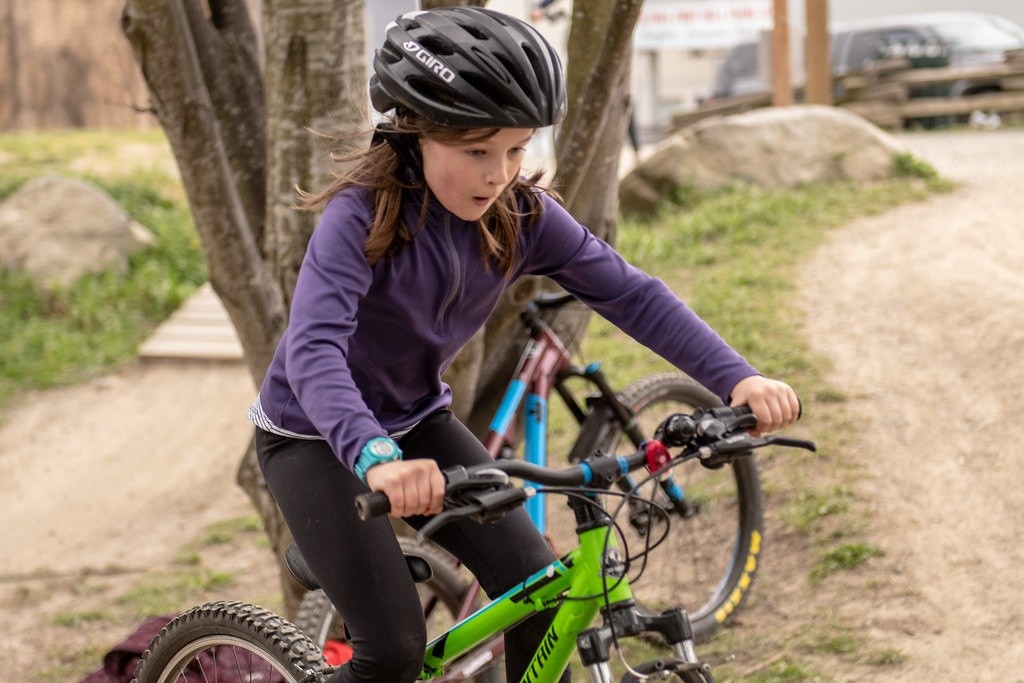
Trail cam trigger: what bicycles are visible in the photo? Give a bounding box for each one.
[127,394,816,683]
[285,276,767,683]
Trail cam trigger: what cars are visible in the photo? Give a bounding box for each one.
[699,10,1024,134]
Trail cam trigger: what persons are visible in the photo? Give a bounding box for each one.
[245,6,800,683]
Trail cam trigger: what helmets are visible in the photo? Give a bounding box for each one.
[370,6,564,129]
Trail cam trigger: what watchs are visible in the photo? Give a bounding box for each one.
[354,435,404,486]
